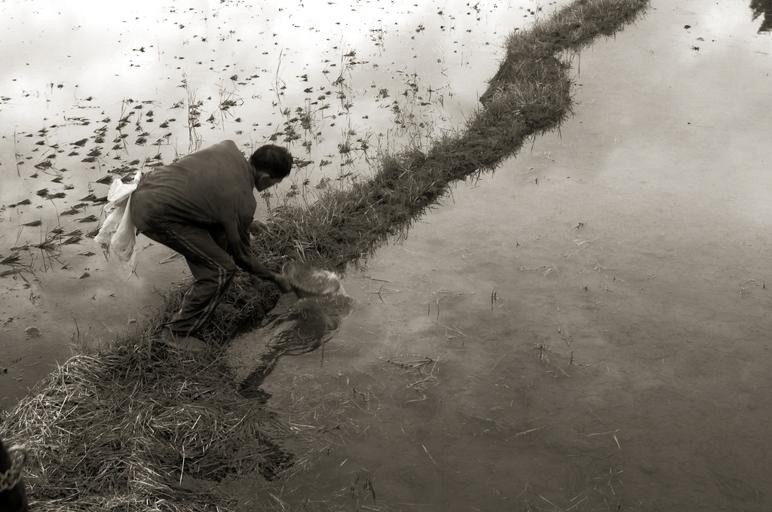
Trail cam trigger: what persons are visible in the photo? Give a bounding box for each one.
[131,140,292,353]
